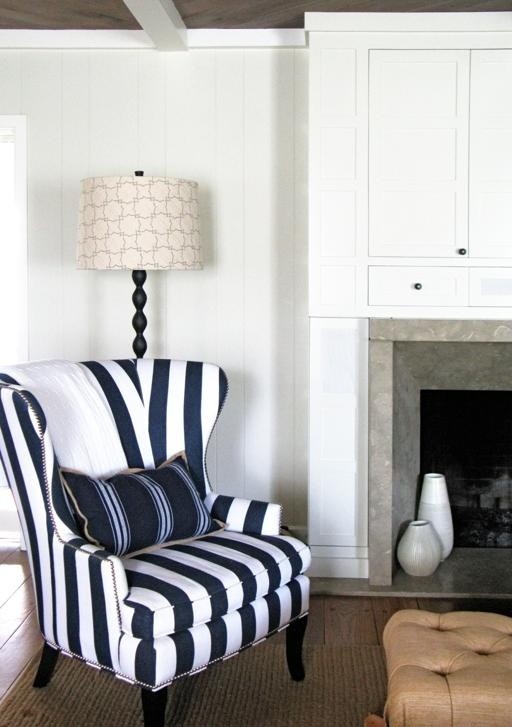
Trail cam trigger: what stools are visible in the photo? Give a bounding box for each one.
[363,609,512,727]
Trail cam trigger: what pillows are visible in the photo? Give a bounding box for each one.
[56,452,228,558]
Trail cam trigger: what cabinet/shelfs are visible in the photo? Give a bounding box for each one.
[305,11,510,320]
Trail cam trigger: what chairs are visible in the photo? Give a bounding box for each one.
[0,359,313,726]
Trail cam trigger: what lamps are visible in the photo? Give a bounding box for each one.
[74,169,206,358]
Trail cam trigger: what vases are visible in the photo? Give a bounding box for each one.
[397,471,457,578]
